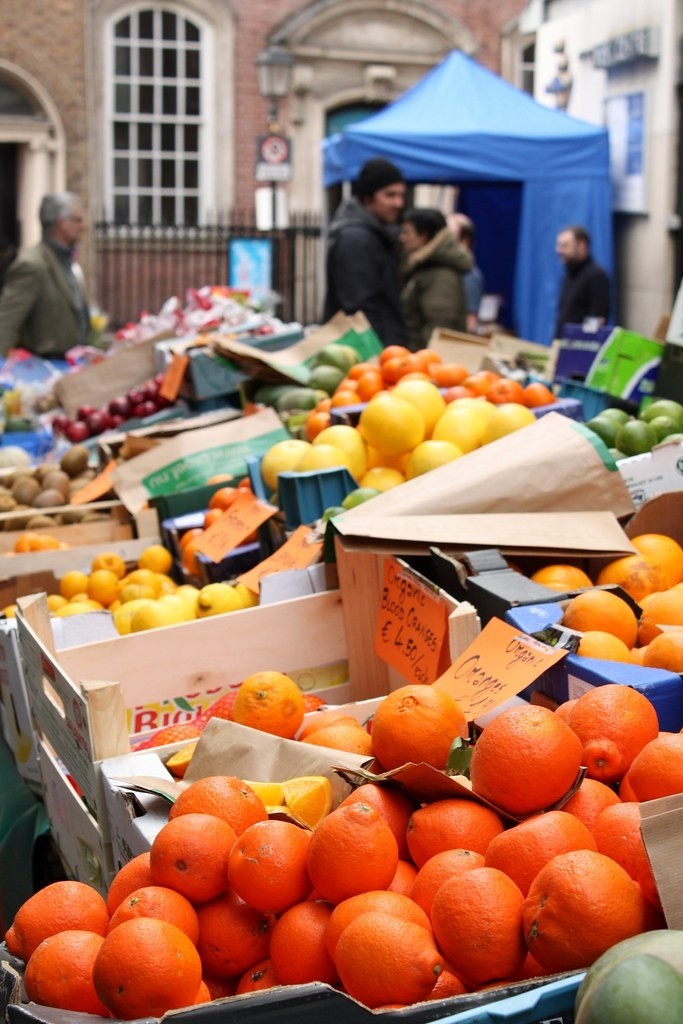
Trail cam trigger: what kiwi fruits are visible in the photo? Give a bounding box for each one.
[0,448,113,530]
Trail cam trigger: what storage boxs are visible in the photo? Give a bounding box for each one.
[0,309,683,1024]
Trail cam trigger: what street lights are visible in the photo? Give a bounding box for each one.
[259,31,291,231]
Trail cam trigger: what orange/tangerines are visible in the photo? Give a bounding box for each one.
[181,475,260,574]
[5,532,683,1020]
[306,347,553,445]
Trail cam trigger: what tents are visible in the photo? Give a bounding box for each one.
[321,50,609,348]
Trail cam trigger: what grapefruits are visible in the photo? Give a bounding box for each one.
[261,377,536,491]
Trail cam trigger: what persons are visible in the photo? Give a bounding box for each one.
[443,212,485,333]
[555,226,610,339]
[325,160,411,348]
[399,207,472,352]
[0,192,93,363]
